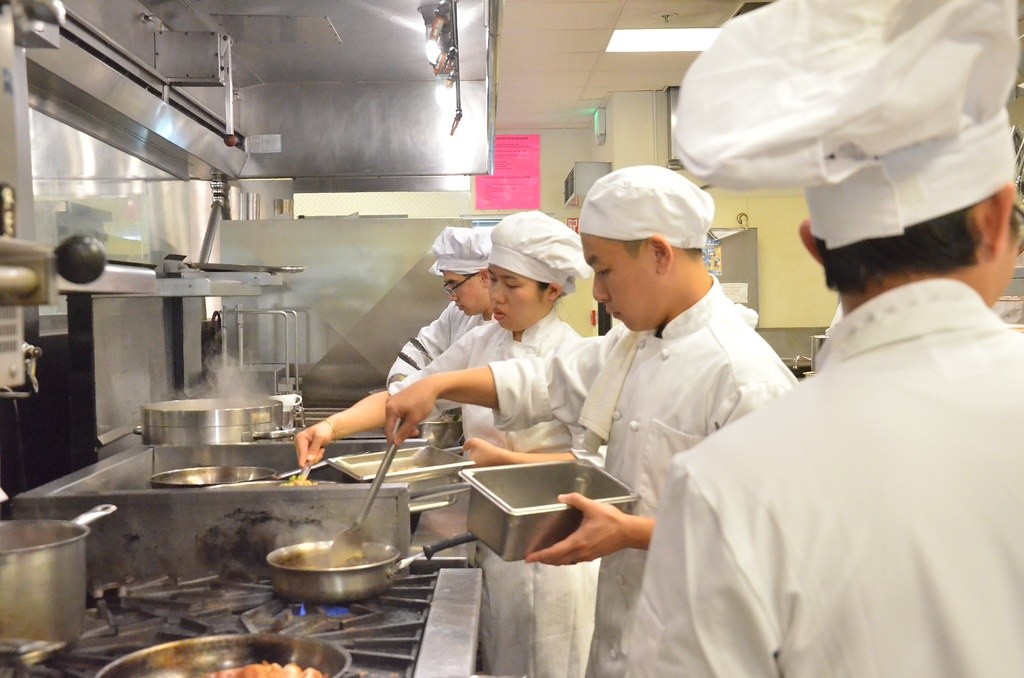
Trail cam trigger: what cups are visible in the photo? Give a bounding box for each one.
[268,393,303,411]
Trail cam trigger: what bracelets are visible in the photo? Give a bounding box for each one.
[322,418,336,445]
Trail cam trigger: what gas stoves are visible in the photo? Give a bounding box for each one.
[0,565,481,678]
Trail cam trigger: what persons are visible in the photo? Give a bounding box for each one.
[383,163,800,678]
[386,226,498,390]
[625,0,1024,677]
[292,209,607,678]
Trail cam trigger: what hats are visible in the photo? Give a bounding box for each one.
[672,0,1021,250]
[577,164,714,249]
[487,211,591,298]
[428,225,495,280]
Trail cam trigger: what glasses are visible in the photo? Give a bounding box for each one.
[441,273,477,296]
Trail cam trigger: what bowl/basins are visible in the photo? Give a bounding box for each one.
[420,421,464,448]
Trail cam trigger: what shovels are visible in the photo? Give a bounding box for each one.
[323,413,411,566]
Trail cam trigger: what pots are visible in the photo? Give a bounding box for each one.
[96,634,352,678]
[266,531,476,604]
[0,504,118,666]
[150,450,372,488]
[133,397,283,444]
[208,480,339,489]
